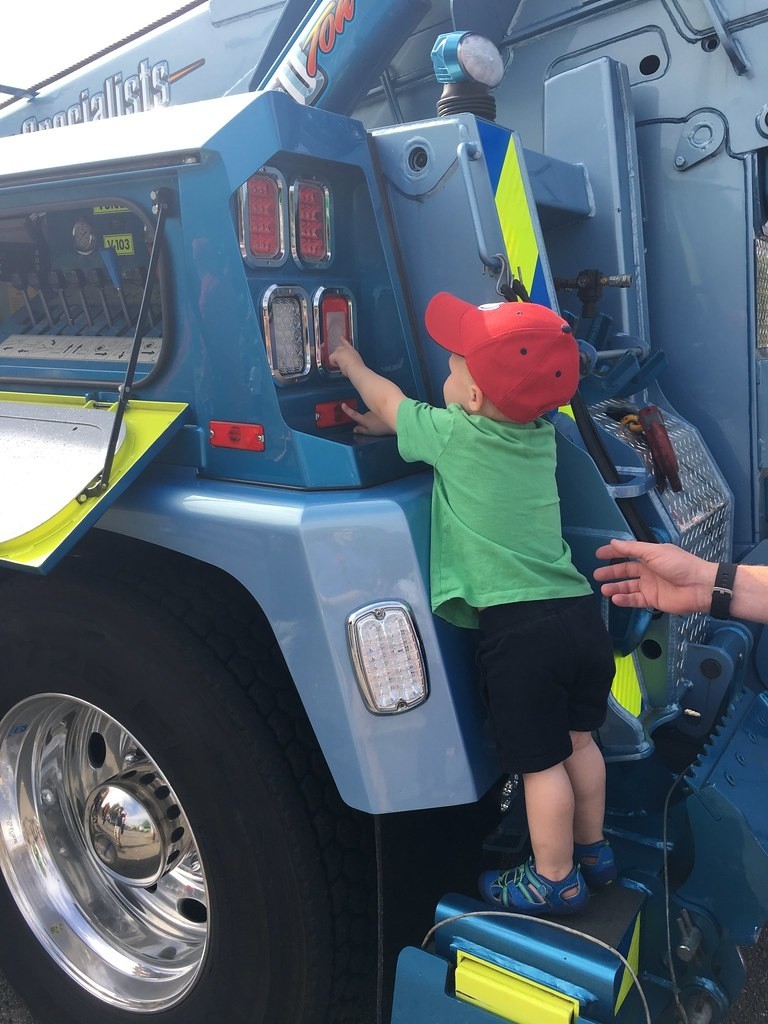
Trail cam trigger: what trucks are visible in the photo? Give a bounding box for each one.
[0,0,768,1024]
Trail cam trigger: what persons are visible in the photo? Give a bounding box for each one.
[328,290,617,915]
[593,538,768,624]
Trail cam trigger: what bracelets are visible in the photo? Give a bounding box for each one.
[708,562,737,621]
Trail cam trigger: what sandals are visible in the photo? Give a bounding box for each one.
[574,840,616,891]
[478,855,590,917]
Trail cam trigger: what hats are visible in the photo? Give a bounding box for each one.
[425,292,580,423]
